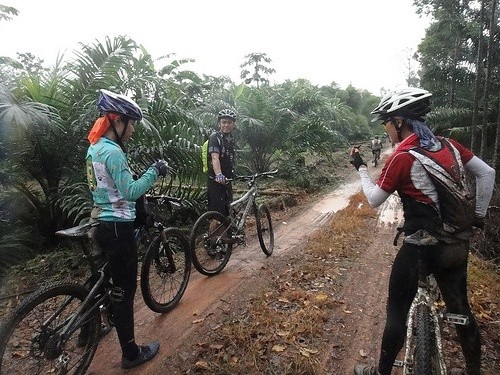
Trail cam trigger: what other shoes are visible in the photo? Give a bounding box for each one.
[78,324,111,347]
[121,341,160,367]
[354,363,380,375]
[208,251,226,260]
[451,367,470,375]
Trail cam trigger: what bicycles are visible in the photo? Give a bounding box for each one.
[0,194,192,375]
[371,147,381,167]
[392,225,470,375]
[190,168,279,276]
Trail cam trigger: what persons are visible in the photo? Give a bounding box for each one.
[78,90,168,369]
[370,135,383,163]
[202,109,245,259]
[349,88,496,375]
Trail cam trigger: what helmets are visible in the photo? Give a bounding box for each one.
[218,109,237,122]
[98,88,143,121]
[370,87,433,123]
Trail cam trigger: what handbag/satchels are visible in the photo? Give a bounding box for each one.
[136,197,147,229]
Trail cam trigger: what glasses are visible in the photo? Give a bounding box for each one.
[380,118,395,125]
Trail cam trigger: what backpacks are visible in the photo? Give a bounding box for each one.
[409,138,474,234]
[200,139,209,174]
